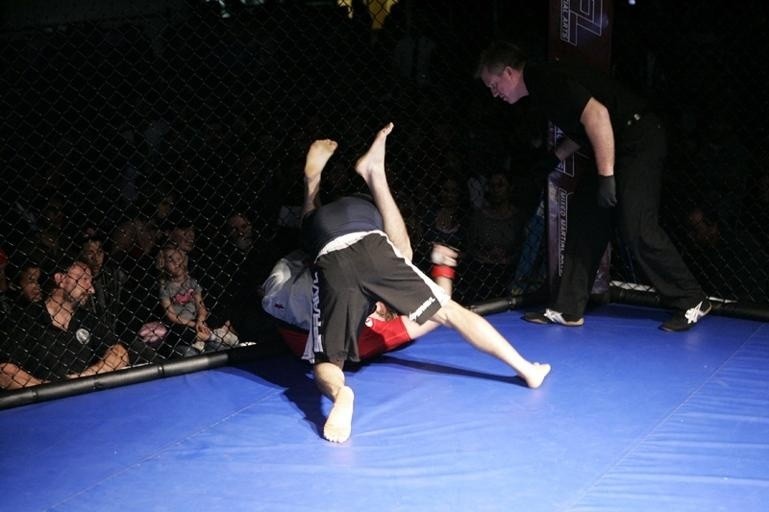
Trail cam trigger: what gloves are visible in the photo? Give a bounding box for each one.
[535,151,561,174]
[595,175,617,207]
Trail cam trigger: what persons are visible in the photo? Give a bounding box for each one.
[481,42,713,334]
[259,122,457,366]
[677,203,731,296]
[301,191,550,443]
[419,173,530,300]
[1,194,259,392]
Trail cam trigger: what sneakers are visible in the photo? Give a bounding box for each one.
[215,326,239,347]
[661,297,712,332]
[524,308,584,326]
